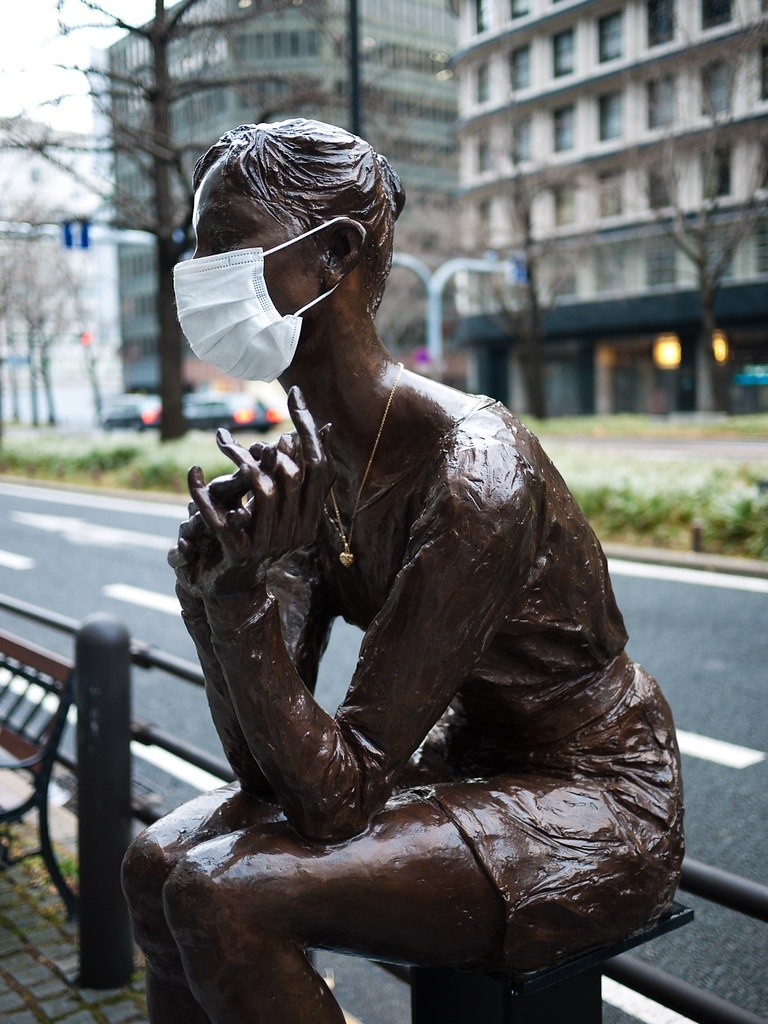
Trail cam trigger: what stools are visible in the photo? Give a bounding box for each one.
[372,901,694,1024]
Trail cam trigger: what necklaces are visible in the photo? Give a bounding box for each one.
[331,363,404,568]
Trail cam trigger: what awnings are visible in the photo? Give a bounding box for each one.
[452,281,768,350]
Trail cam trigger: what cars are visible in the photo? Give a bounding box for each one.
[96,387,281,435]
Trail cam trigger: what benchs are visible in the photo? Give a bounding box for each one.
[0,661,80,924]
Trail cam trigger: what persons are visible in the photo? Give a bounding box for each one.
[121,117,686,1024]
